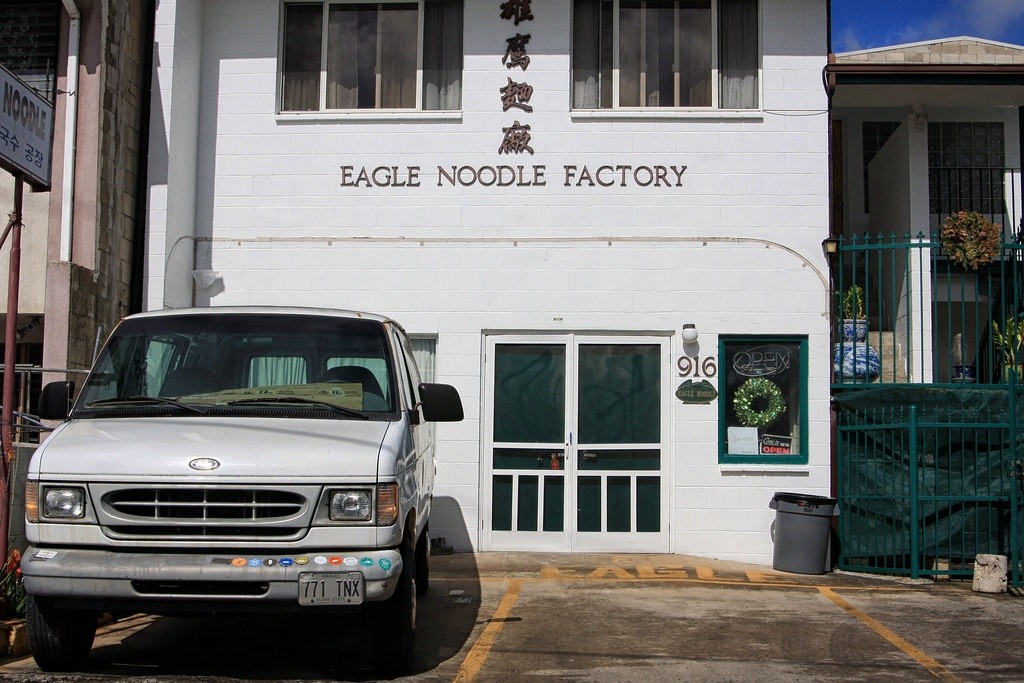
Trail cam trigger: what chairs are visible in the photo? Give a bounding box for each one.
[319,366,385,398]
[157,368,234,398]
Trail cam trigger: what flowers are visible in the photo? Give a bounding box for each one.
[0,535,28,618]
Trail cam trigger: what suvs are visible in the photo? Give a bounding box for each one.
[21,303,466,674]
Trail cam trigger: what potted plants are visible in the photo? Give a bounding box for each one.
[834,283,881,384]
[952,333,976,383]
[991,316,1024,384]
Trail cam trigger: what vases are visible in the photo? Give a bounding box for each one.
[0,617,30,661]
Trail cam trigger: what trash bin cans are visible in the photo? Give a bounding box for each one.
[769,492,841,575]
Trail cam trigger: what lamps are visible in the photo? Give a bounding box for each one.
[681,323,698,342]
[192,270,221,291]
[823,231,840,254]
